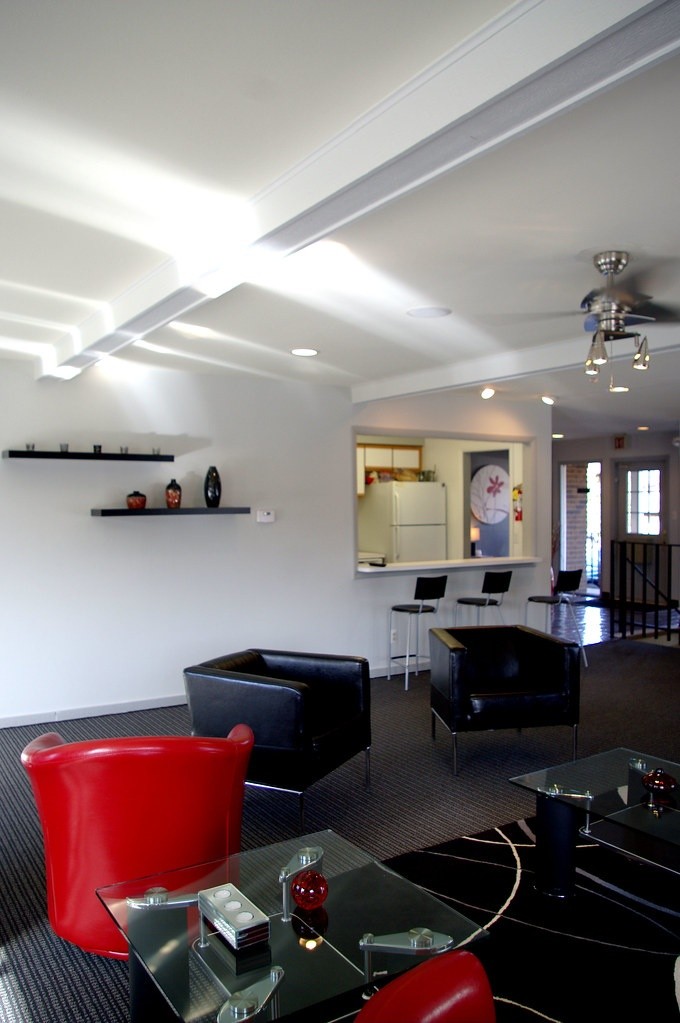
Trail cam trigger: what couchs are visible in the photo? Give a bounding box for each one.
[428,626,584,778]
[20,722,254,961]
[183,649,371,830]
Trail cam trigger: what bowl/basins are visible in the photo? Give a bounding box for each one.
[418,470,434,481]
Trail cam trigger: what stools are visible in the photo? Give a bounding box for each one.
[524,568,588,667]
[386,575,448,690]
[456,570,512,626]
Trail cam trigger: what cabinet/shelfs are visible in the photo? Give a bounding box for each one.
[357,445,424,497]
[7,450,252,517]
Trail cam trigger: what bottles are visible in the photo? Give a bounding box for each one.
[204,467,221,508]
[165,479,182,508]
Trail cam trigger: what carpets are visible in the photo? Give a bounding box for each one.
[277,807,680,1023]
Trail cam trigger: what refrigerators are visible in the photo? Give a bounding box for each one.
[358,481,446,562]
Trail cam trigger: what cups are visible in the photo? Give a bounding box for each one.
[120,446,129,454]
[153,447,160,454]
[126,491,146,508]
[26,442,34,449]
[60,444,68,452]
[641,767,677,816]
[93,445,101,452]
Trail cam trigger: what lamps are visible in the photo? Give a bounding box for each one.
[481,387,495,400]
[541,396,557,405]
[585,328,650,392]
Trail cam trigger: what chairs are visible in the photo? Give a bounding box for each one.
[354,950,494,1023]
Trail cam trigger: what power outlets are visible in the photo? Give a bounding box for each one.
[392,631,398,644]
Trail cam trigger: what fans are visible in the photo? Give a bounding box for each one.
[454,248,680,328]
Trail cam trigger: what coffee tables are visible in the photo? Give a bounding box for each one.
[509,748,680,899]
[93,829,491,1023]
